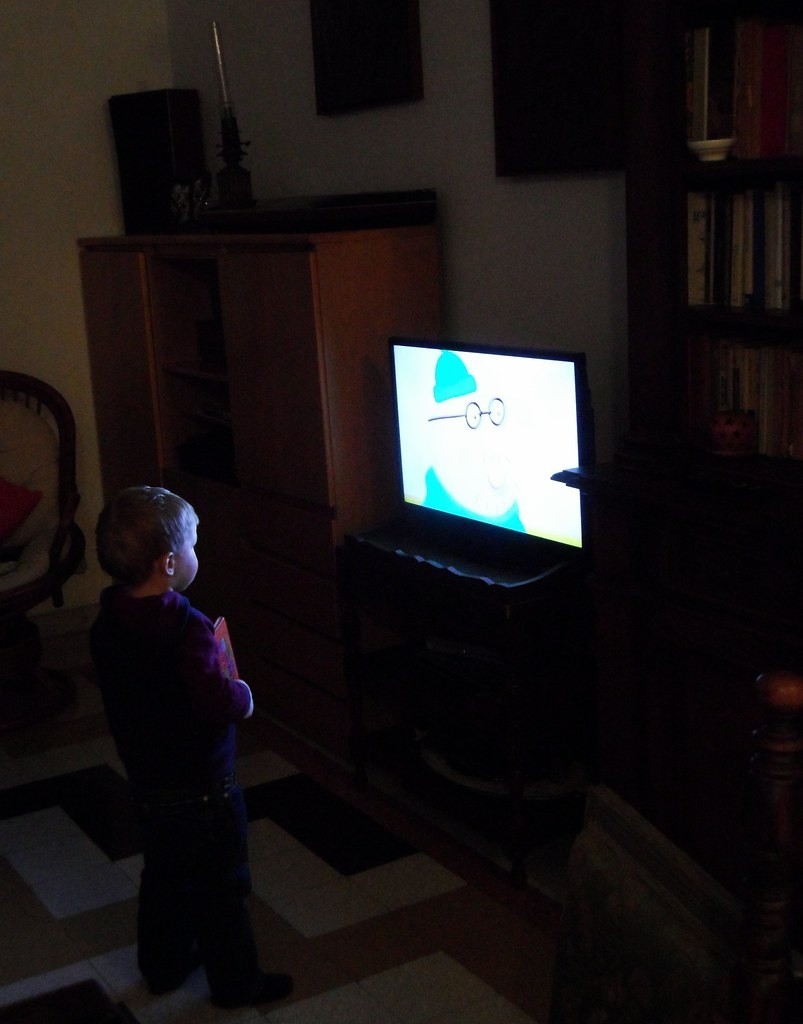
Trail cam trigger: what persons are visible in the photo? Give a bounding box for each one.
[87,488,294,1010]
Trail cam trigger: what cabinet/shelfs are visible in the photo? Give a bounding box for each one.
[78,220,444,773]
[338,517,592,858]
[578,462,803,956]
[615,1,803,487]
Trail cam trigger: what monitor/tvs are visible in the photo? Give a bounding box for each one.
[388,335,592,555]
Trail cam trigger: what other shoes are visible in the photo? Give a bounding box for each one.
[135,950,200,994]
[209,970,294,1009]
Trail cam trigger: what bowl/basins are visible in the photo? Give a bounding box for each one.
[688,139,736,161]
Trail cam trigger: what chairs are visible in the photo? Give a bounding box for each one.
[0,370,86,711]
[536,779,747,1024]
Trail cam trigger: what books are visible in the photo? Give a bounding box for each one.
[684,19,802,158]
[687,335,803,459]
[688,183,791,310]
